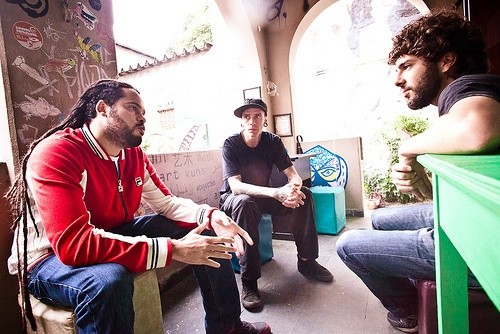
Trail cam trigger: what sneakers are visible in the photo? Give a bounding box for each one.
[240,279,262,310]
[224,319,271,334]
[296,254,334,282]
[386,309,420,334]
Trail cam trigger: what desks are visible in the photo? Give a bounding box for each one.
[269,152,323,241]
[416,153,500,334]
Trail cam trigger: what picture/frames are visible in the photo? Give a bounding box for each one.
[243,86,261,101]
[273,113,293,137]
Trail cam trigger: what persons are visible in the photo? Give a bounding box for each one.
[219,99,333,309]
[337,7,500,332]
[5,79,272,334]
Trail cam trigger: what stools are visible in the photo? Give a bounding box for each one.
[311,186,346,235]
[416,280,500,334]
[230,213,274,273]
[18,269,164,334]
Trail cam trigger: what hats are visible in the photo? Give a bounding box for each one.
[233,98,268,118]
